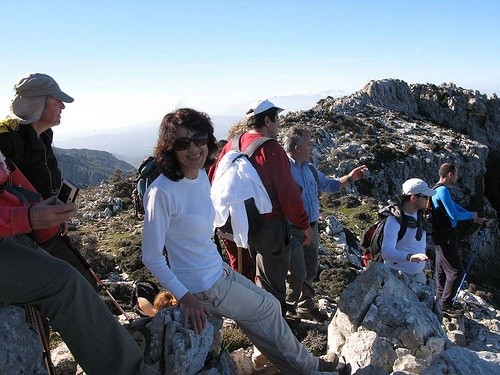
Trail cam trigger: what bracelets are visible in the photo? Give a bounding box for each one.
[178,291,191,302]
[345,175,354,186]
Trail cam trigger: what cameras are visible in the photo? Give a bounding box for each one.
[54,179,80,206]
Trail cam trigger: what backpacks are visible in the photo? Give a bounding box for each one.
[213,133,278,234]
[361,211,423,266]
[423,198,432,233]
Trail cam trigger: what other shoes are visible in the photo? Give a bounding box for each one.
[296,306,328,321]
[441,302,465,317]
[317,351,346,374]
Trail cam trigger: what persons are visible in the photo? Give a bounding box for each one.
[205,99,371,322]
[0,73,152,375]
[382,177,437,285]
[142,108,340,375]
[423,162,493,317]
[130,273,178,318]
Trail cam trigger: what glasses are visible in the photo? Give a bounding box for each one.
[172,130,210,150]
[418,195,428,199]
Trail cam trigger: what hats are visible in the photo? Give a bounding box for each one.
[245,99,285,119]
[400,177,437,196]
[8,73,75,124]
